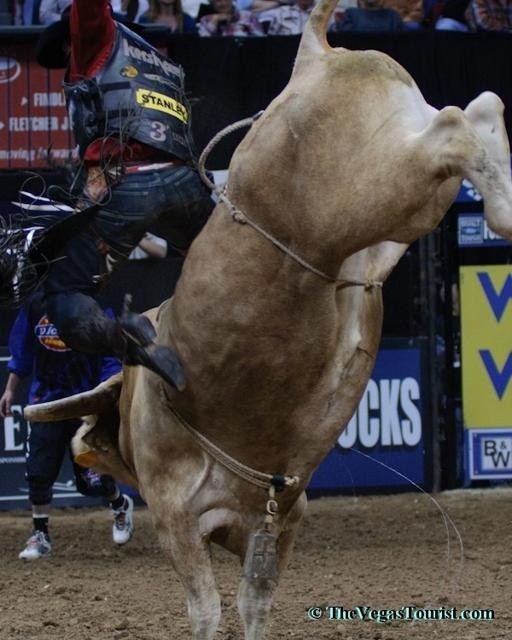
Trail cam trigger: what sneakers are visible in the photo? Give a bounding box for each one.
[109,493,134,543]
[18,532,53,562]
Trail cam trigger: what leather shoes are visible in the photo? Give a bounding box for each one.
[112,315,186,393]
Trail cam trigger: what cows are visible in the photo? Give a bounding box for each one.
[23,0,512,640]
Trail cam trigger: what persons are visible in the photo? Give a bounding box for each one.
[1,306,135,563]
[20,1,219,393]
[39,2,511,40]
